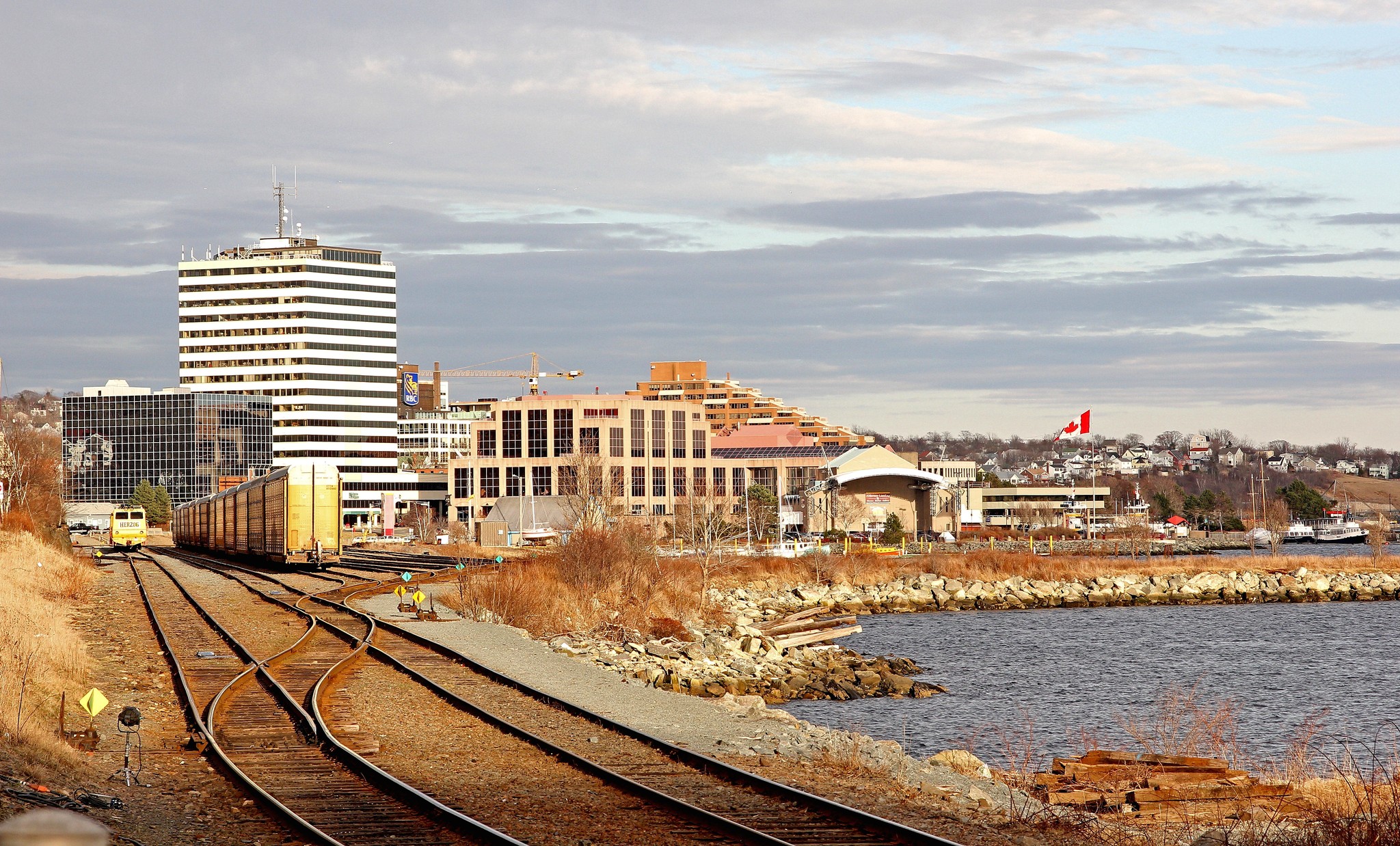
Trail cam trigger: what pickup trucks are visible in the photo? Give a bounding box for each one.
[68,523,99,535]
[917,530,956,541]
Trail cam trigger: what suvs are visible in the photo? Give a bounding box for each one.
[1075,531,1086,539]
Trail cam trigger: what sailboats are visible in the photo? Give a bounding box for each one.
[645,465,833,560]
[516,468,559,539]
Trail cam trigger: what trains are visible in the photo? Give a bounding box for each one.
[171,461,343,566]
[109,503,148,551]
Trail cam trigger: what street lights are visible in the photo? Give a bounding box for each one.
[1002,509,1009,530]
[512,474,523,549]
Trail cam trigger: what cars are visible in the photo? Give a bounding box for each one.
[853,533,867,539]
[103,527,110,533]
[351,534,420,545]
[395,521,407,527]
[912,534,934,542]
[849,534,864,539]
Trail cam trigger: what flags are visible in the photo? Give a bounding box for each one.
[1053,408,1091,442]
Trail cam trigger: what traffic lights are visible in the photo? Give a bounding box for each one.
[367,516,369,521]
[430,508,432,513]
[367,511,370,516]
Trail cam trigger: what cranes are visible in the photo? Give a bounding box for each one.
[419,352,585,396]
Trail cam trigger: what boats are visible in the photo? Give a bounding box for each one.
[1062,478,1151,533]
[1244,458,1371,545]
[851,544,903,558]
